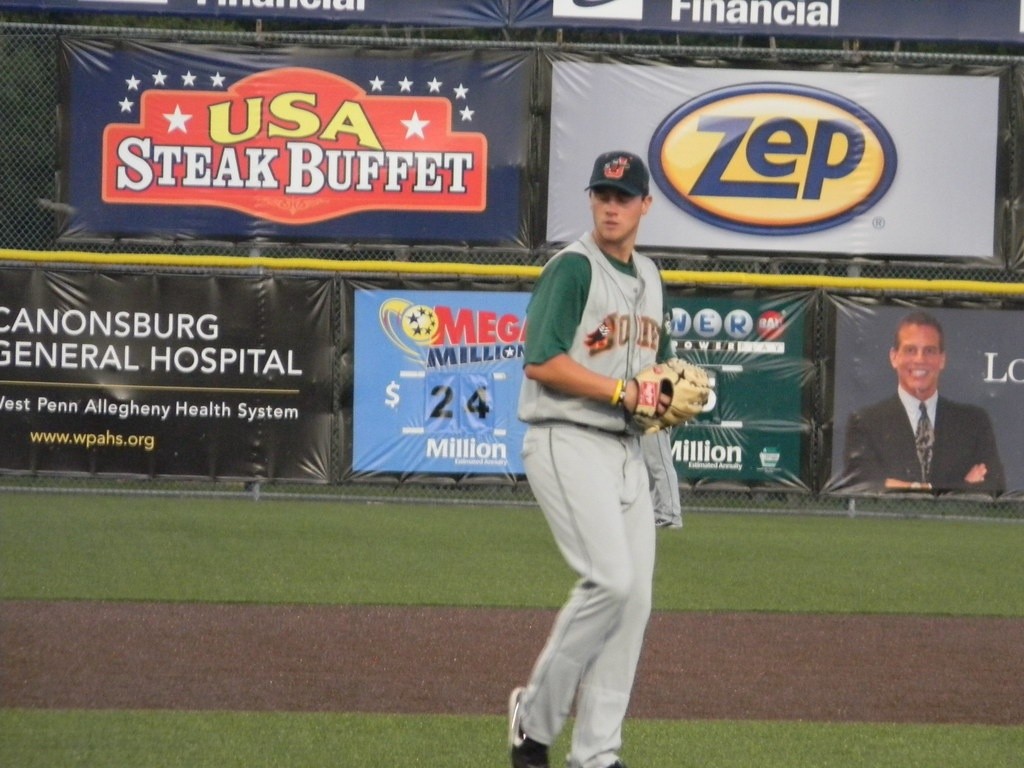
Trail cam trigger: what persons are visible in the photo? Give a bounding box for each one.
[840,312,1008,501]
[640,423,683,534]
[504,152,666,768]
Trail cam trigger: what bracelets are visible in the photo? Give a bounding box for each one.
[610,376,623,408]
[616,379,628,412]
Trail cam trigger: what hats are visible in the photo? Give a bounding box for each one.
[584,150,650,196]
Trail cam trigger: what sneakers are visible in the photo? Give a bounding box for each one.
[506,685,549,768]
[655,518,682,528]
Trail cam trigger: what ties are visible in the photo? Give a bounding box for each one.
[915,402,933,481]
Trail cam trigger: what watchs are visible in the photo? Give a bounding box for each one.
[908,480,921,491]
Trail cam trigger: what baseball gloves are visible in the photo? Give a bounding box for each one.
[631,358,710,433]
